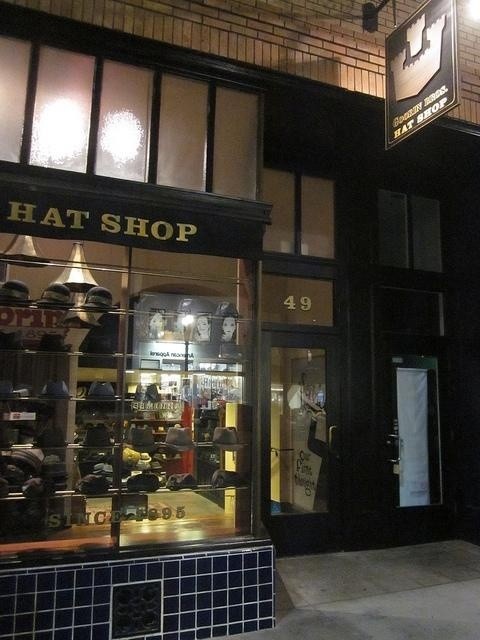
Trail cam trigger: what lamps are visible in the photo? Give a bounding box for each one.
[48,240,100,292]
[0,234,50,267]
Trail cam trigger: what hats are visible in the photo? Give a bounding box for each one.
[0,280,120,400]
[1,425,245,492]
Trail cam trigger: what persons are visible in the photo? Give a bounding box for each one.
[219,317,236,341]
[149,312,162,333]
[195,315,210,341]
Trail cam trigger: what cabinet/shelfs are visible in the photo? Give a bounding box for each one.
[0,295,251,501]
